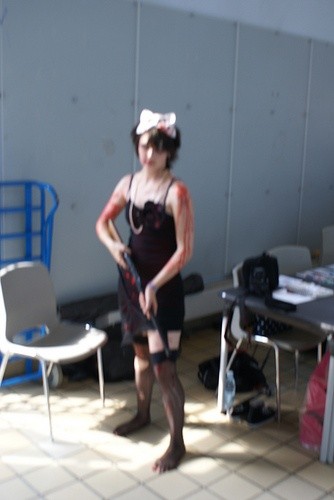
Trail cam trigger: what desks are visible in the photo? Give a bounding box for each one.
[216,266,334,414]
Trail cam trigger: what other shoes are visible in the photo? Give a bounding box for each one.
[248,399,278,431]
[232,401,255,429]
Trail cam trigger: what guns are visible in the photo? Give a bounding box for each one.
[105,219,175,362]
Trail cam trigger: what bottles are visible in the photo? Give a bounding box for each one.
[11,322,50,345]
[221,369,236,412]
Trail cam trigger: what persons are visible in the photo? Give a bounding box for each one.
[96,109,195,474]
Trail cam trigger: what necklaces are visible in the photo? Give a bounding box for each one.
[129,169,168,235]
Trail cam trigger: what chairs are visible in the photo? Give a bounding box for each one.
[0,261,105,442]
[231,226,334,424]
